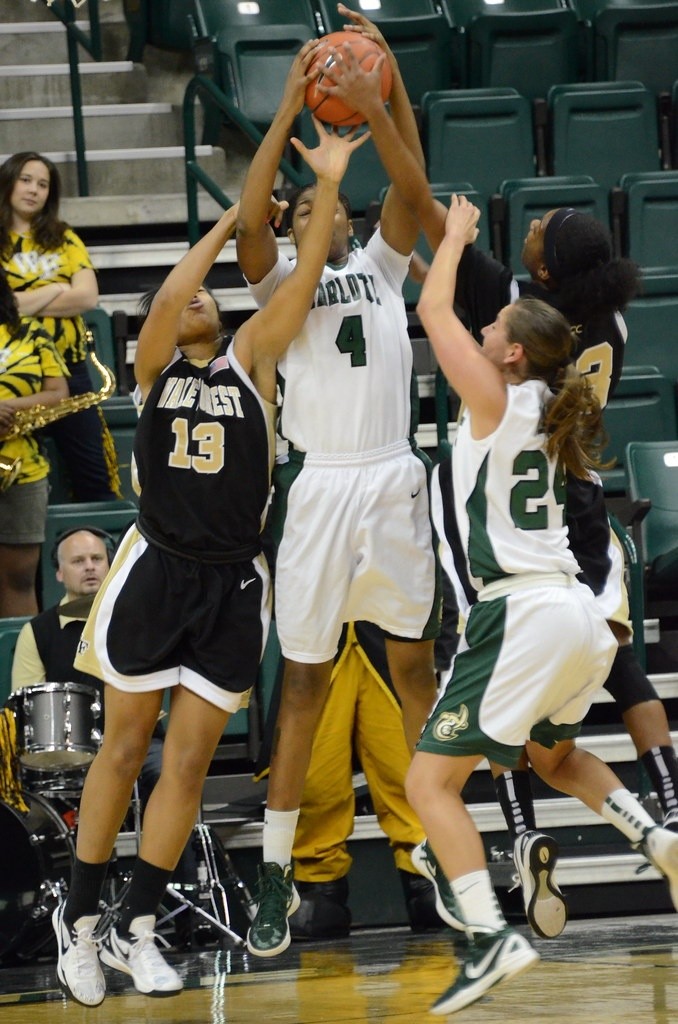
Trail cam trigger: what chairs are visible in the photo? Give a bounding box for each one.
[0,0,678,751]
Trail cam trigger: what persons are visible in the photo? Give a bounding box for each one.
[52,0,678,1014]
[11,527,213,953]
[0,270,70,618]
[0,150,131,506]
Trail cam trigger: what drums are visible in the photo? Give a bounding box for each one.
[162,823,229,892]
[22,768,87,799]
[0,788,76,961]
[13,682,100,773]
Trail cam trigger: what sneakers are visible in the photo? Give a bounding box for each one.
[430,924,542,1018]
[645,824,677,909]
[52,895,107,1008]
[511,829,567,940]
[246,861,301,957]
[411,836,466,933]
[99,912,185,999]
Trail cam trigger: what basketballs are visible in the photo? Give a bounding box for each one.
[304,29,394,128]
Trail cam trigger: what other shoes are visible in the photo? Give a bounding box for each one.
[639,797,678,830]
